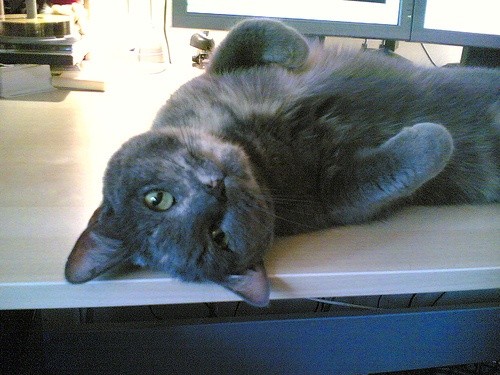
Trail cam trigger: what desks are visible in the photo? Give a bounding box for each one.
[1,59,499,375]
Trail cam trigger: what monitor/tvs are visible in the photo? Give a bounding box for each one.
[172,0,414,50]
[406,0,500,67]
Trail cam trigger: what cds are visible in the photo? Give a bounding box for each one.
[0,13,75,39]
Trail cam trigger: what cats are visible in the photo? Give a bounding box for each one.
[64,18,500,307]
